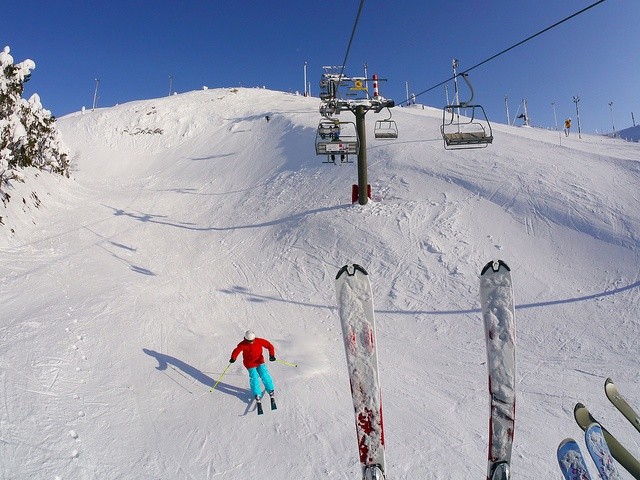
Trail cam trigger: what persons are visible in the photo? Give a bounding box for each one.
[330,134,345,166]
[229,330,277,415]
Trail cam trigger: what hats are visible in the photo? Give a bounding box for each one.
[244,329,256,341]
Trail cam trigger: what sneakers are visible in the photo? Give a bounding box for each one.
[255,394,261,403]
[269,391,274,399]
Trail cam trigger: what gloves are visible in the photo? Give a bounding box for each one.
[229,357,236,363]
[269,354,276,361]
